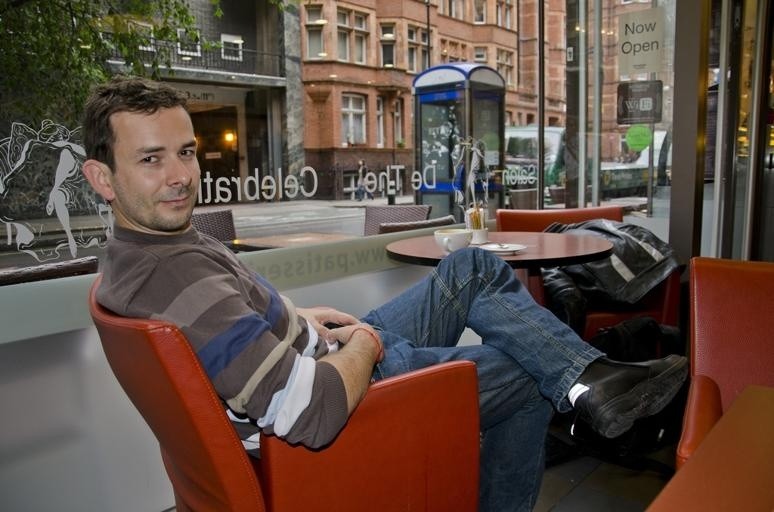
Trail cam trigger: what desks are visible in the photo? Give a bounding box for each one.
[223,232,362,252]
[385,231,614,463]
[645,384,773,510]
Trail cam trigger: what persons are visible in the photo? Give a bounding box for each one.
[82,76,688,512]
[356,159,374,202]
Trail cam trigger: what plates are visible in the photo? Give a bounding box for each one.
[480,243,526,256]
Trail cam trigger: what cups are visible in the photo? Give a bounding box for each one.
[434,229,473,256]
[470,229,488,245]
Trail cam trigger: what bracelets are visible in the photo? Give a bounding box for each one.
[348,327,383,362]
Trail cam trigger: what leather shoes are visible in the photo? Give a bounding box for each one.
[568,352,689,438]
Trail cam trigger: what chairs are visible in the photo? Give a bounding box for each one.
[0,255,98,287]
[496,207,679,345]
[90,273,479,512]
[190,209,236,243]
[364,205,432,237]
[677,255,773,471]
[378,214,456,234]
